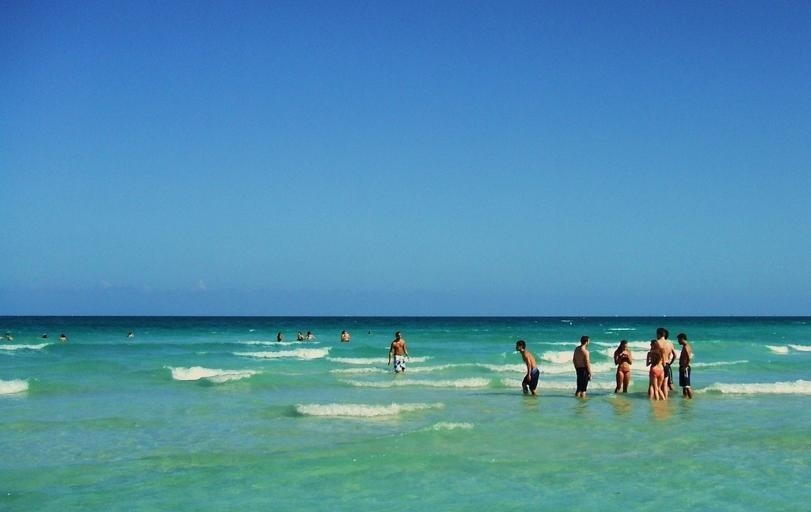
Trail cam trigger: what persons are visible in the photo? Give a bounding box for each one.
[277,328,351,343]
[614,340,633,393]
[572,336,593,398]
[645,328,694,400]
[388,331,409,374]
[515,340,539,395]
